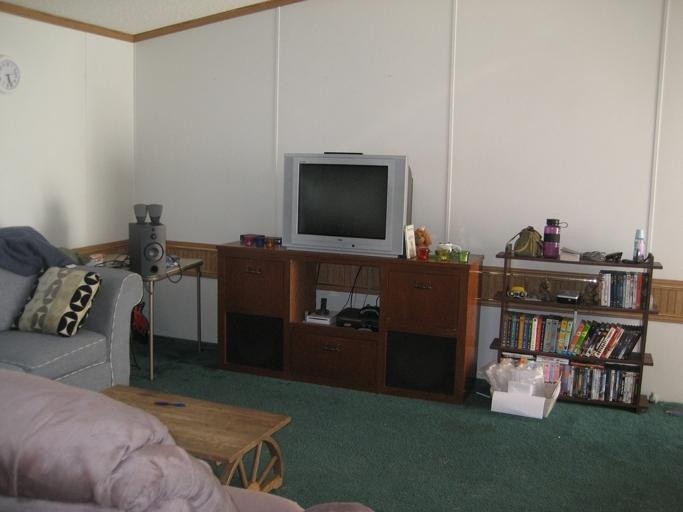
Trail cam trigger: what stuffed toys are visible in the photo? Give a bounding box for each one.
[415,228,432,260]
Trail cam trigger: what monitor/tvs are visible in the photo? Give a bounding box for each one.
[282,154,414,259]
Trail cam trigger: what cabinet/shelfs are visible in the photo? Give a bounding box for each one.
[215,238,484,406]
[491,243,663,413]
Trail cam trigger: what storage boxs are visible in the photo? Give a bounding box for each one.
[486,364,561,420]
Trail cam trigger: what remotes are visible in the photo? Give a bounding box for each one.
[325,151,364,155]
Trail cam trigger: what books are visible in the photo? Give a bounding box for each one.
[403,224,419,259]
[501,311,642,406]
[594,270,644,312]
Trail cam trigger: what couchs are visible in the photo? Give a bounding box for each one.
[0,368,373,511]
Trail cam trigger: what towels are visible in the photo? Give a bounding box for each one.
[0,226,77,277]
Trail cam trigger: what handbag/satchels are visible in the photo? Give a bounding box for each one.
[514,226,543,258]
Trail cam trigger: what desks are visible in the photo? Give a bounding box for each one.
[100,254,204,380]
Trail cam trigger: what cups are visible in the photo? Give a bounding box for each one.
[417,248,469,263]
[243,235,275,248]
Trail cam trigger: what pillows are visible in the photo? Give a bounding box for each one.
[10,265,104,338]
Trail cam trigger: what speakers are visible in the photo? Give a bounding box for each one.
[127,222,166,277]
[148,204,163,225]
[134,203,148,223]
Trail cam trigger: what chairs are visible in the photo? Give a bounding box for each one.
[0,226,144,396]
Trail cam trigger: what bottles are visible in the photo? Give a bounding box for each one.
[634,229,647,263]
[542,218,562,259]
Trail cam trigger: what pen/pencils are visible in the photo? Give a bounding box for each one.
[155,401,187,407]
